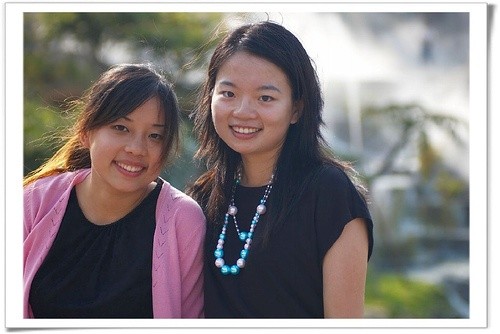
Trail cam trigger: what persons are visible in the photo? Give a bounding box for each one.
[23,64,206,319]
[182,13,374,319]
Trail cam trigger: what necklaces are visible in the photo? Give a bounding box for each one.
[214,156,278,274]
